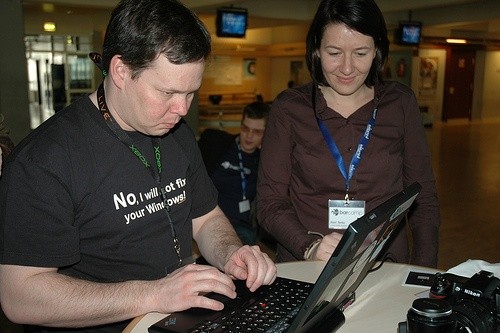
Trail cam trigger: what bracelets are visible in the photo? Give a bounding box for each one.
[306,236,322,260]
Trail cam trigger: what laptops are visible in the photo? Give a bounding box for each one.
[149,181,423,333]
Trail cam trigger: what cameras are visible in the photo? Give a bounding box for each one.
[398,269,500,333]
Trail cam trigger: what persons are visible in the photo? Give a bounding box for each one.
[198,102,276,251]
[255,0,440,270]
[0,0,278,333]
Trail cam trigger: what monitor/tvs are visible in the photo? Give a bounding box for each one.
[398,22,422,45]
[216,8,247,37]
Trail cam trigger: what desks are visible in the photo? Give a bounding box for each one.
[121,259,446,333]
